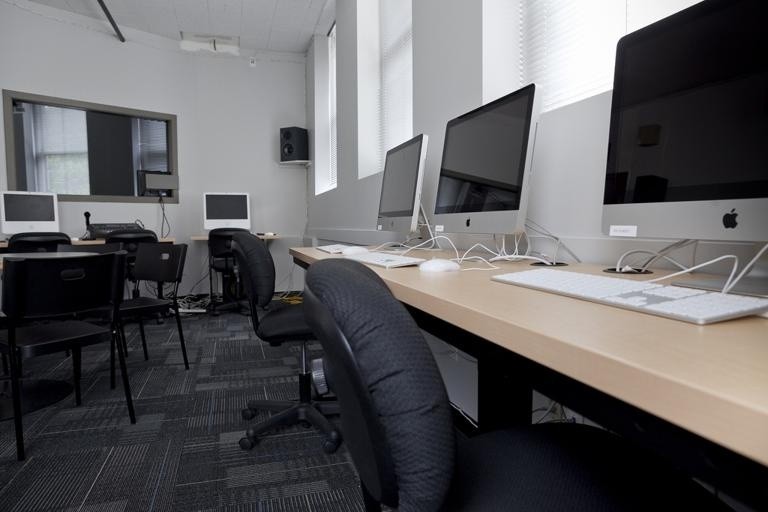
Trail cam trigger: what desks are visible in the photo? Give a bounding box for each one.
[190,233,280,310]
[288,245,768,512]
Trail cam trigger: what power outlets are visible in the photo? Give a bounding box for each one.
[561,407,583,423]
[544,396,566,422]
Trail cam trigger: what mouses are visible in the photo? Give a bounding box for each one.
[419,258,460,273]
[342,245,369,257]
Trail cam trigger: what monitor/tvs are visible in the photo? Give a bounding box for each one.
[432,83,543,238]
[376,134,435,251]
[136,169,172,197]
[204,192,251,231]
[601,0,767,298]
[1,191,59,234]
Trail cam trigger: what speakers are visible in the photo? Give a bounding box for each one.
[280,126,308,162]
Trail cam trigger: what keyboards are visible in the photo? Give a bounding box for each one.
[316,244,344,254]
[490,266,767,326]
[358,252,417,269]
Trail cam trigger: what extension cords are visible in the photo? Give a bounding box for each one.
[169,305,207,315]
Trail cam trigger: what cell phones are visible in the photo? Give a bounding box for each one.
[256,232,264,235]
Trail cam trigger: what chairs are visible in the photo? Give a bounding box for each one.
[207,228,256,317]
[229,231,345,455]
[301,260,734,512]
